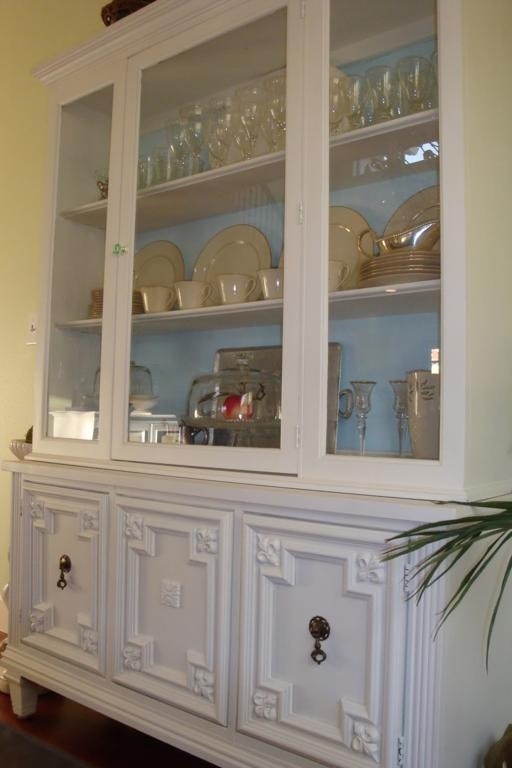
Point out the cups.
[135,258,354,315]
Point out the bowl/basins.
[129,393,158,410]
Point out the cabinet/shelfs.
[0,1,512,762]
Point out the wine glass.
[128,51,431,190]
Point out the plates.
[83,283,142,323]
[119,235,186,318]
[192,221,274,308]
[279,202,376,292]
[357,179,442,291]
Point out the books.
[51,408,181,445]
